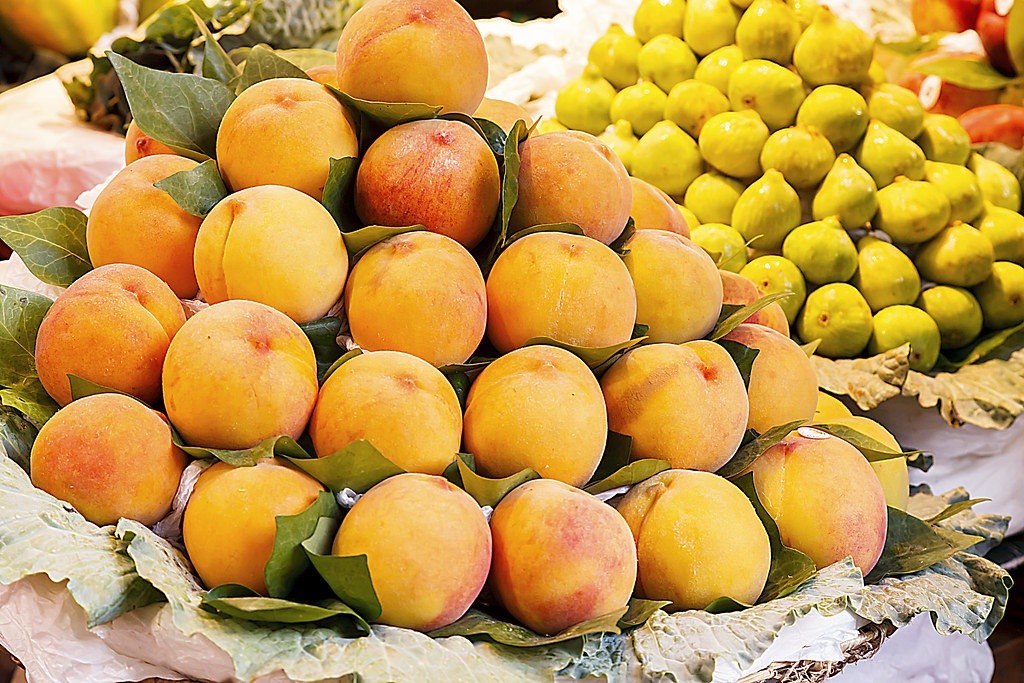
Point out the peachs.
[26,0,908,632]
[894,0,1024,157]
